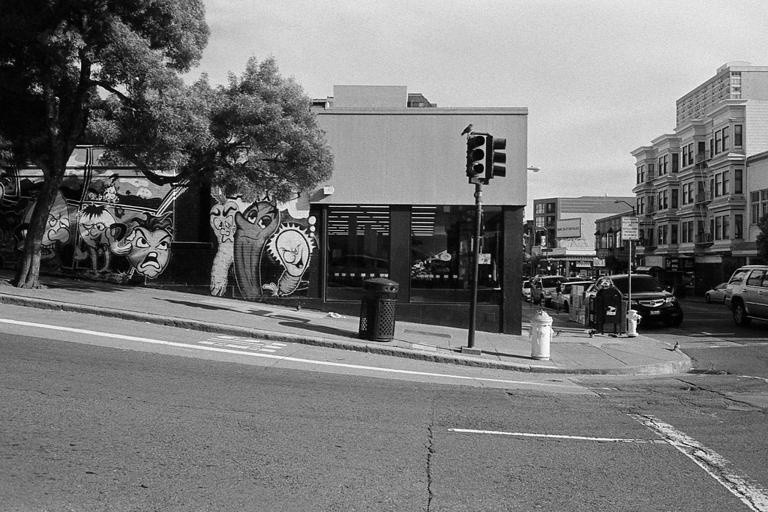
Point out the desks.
[613,199,635,216]
[527,163,541,174]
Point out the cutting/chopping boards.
[528,309,554,360]
[625,310,641,336]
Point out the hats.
[705,282,728,305]
[521,272,683,331]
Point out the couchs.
[466,136,484,179]
[486,133,507,183]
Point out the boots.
[724,265,768,330]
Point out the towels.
[593,284,623,336]
[359,278,400,342]
